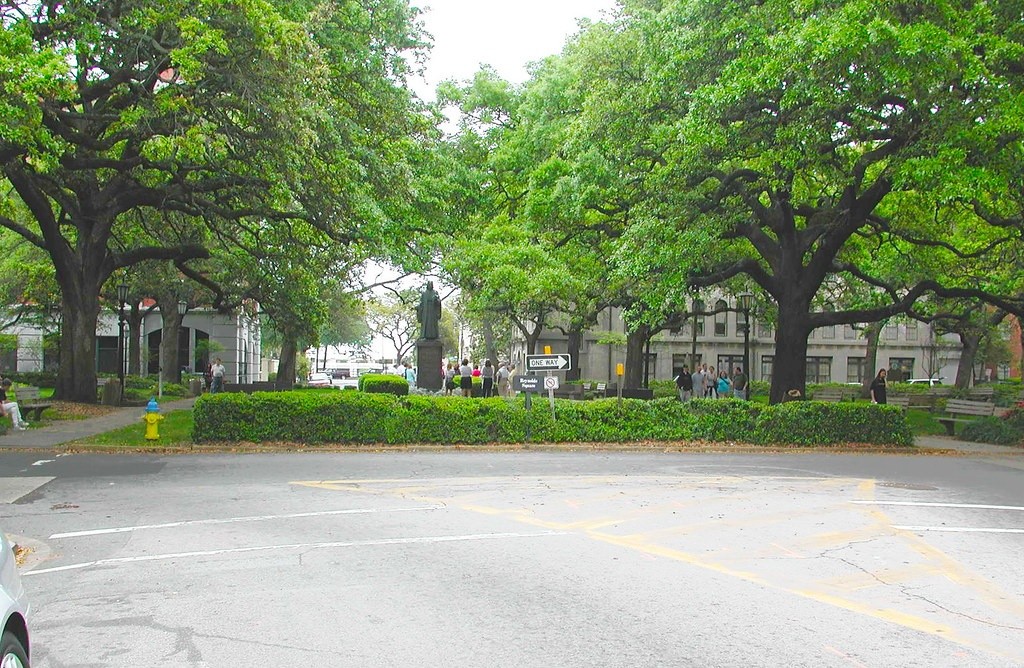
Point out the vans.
[321,368,350,380]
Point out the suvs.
[906,378,942,387]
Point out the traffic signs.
[525,353,572,372]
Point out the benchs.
[582,382,606,398]
[811,388,843,403]
[901,384,993,412]
[935,398,996,436]
[13,386,51,421]
[96,377,107,392]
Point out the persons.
[210,358,226,394]
[871,369,887,404]
[203,363,213,392]
[392,361,416,386]
[733,367,748,401]
[415,281,441,342]
[445,359,518,397]
[0,379,30,431]
[677,363,733,403]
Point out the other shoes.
[14,428,25,431]
[18,423,29,426]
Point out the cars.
[360,369,383,376]
[308,373,330,386]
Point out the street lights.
[117,278,131,393]
[644,320,652,389]
[176,299,188,348]
[740,285,754,401]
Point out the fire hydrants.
[141,396,164,441]
